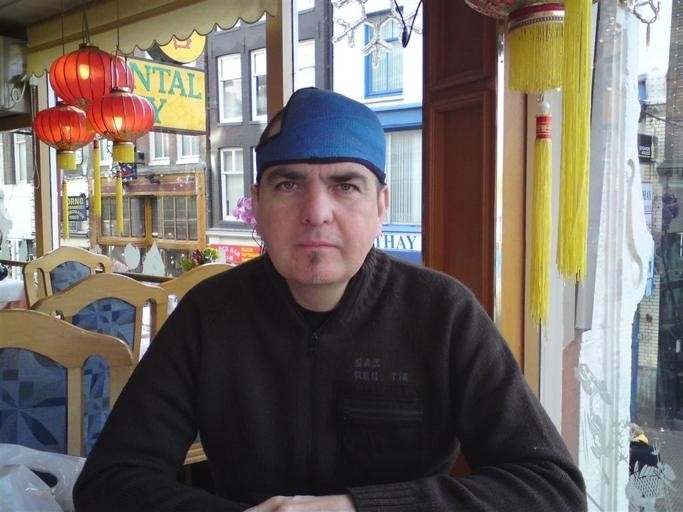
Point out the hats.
[255,87,387,187]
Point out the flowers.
[230,197,265,255]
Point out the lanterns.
[48,42,134,216]
[34,102,93,239]
[87,82,156,234]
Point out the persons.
[628,422,662,512]
[72,86,587,511]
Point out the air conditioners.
[0,36,30,119]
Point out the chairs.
[20,245,111,310]
[156,262,236,306]
[0,308,135,458]
[32,272,169,365]
